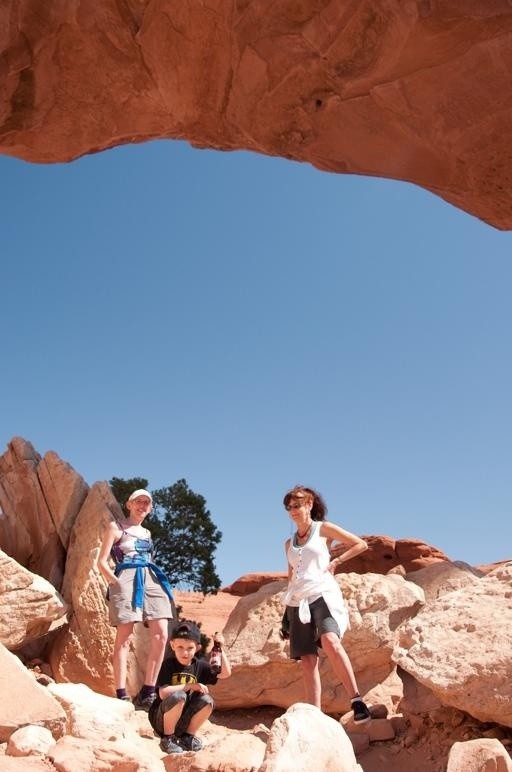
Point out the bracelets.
[330,561,337,568]
[334,557,343,565]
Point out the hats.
[171,622,201,643]
[128,489,153,503]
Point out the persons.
[281,486,371,725]
[149,623,231,754]
[97,488,173,711]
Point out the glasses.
[285,502,307,511]
[132,500,151,505]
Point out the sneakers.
[120,693,156,712]
[159,734,202,755]
[351,696,371,725]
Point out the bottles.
[211,633,222,675]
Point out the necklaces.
[296,519,314,538]
